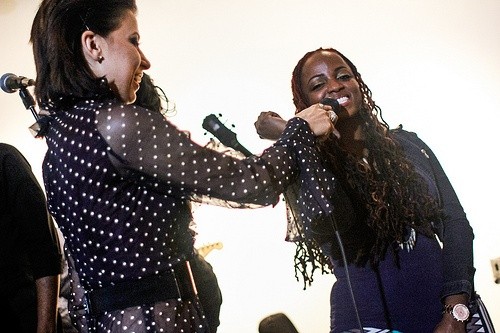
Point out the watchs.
[442,302,472,324]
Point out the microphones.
[0,73,33,93]
[321,98,340,114]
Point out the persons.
[29,1,343,333]
[254,47,494,333]
[0,142,65,333]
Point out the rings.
[319,102,324,109]
[328,109,338,122]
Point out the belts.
[86,258,208,316]
